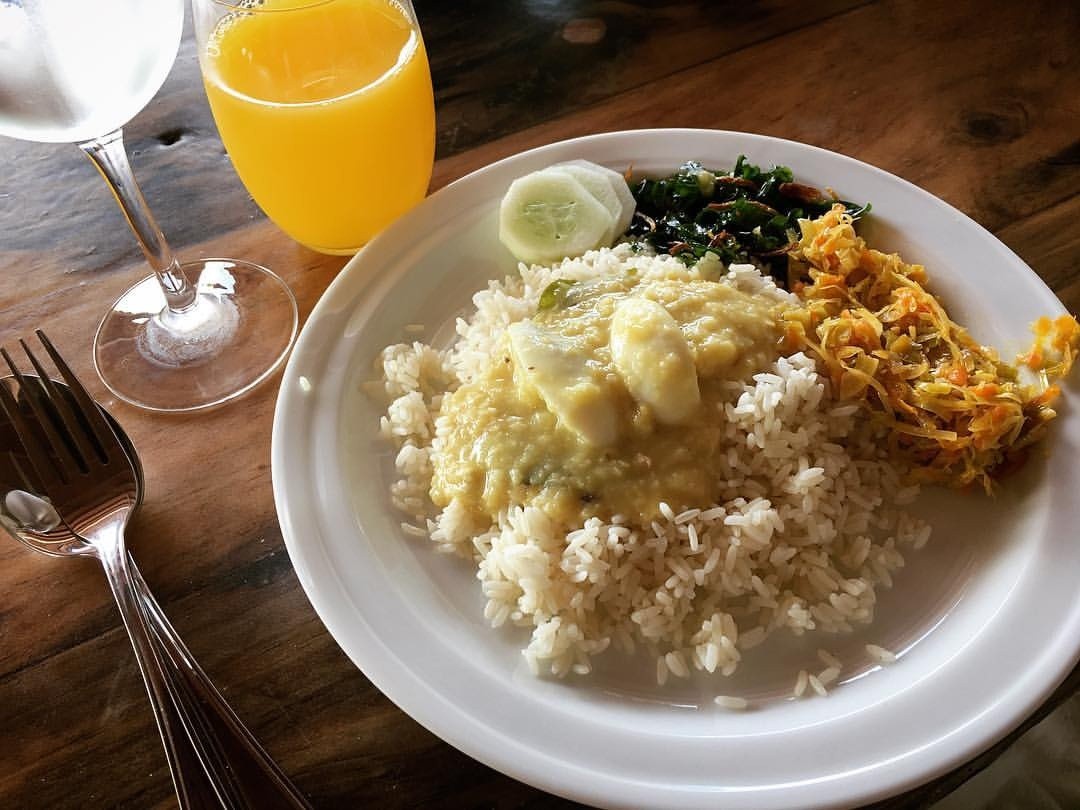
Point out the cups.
[190,0,440,262]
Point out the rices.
[349,223,948,722]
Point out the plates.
[273,124,1080,806]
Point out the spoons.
[0,372,314,810]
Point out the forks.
[0,321,239,808]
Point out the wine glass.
[0,0,299,412]
